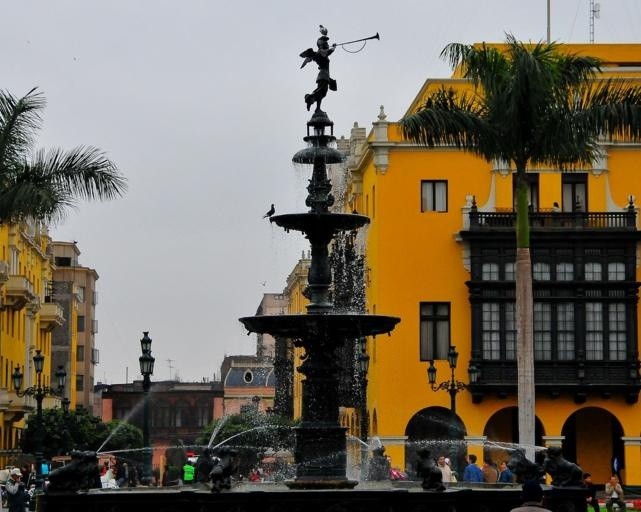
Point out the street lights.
[11,347,67,494]
[138,331,157,482]
[426,344,482,417]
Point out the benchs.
[586,502,634,512]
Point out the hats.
[11,468,23,476]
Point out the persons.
[298,36,337,111]
[369,444,553,489]
[604,474,626,512]
[5,466,30,512]
[583,473,600,512]
[19,464,30,485]
[509,482,554,512]
[98,449,294,489]
